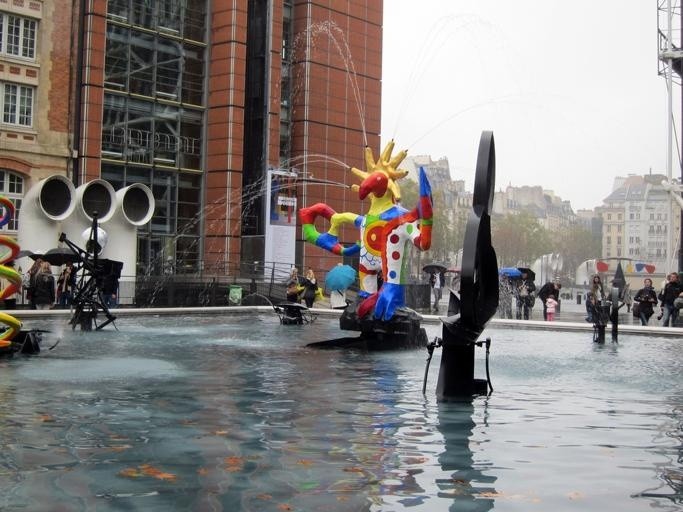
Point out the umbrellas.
[27,254,62,266]
[445,266,461,273]
[43,248,83,263]
[14,250,33,258]
[422,263,447,274]
[324,264,357,290]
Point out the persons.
[278,266,298,289]
[32,261,57,309]
[57,261,78,307]
[329,290,349,310]
[26,258,44,309]
[5,261,24,303]
[498,259,682,328]
[451,273,460,294]
[284,279,304,325]
[303,268,318,309]
[429,267,444,313]
[99,278,119,309]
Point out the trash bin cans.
[228,285,242,306]
